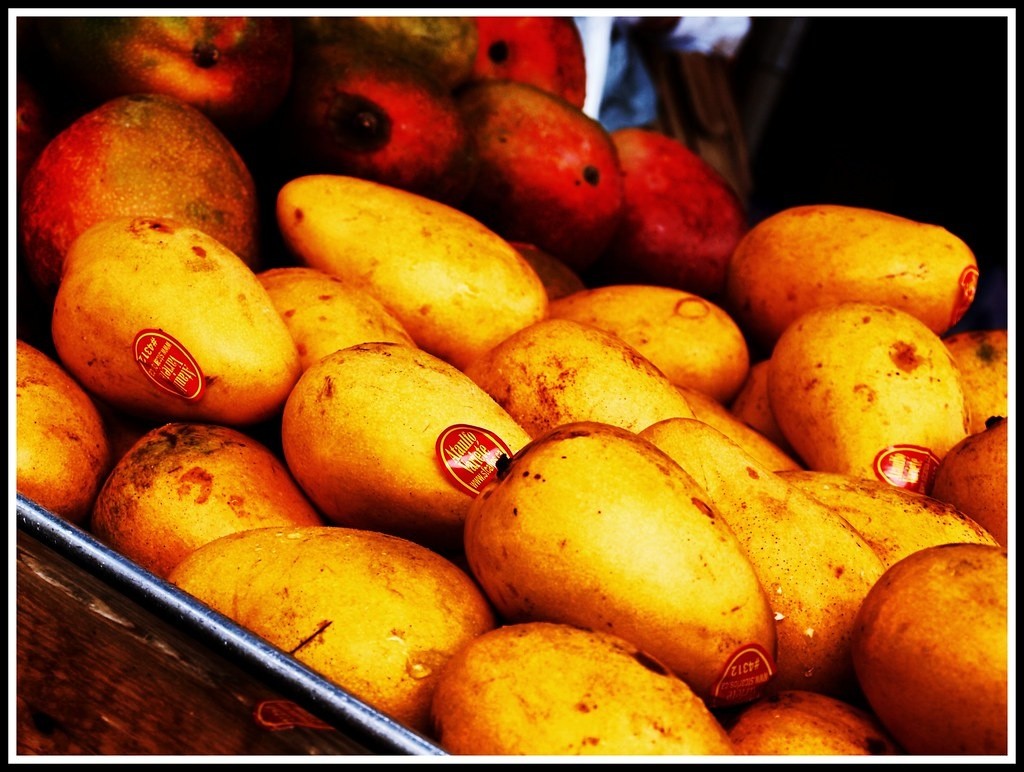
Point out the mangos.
[17,16,1008,755]
